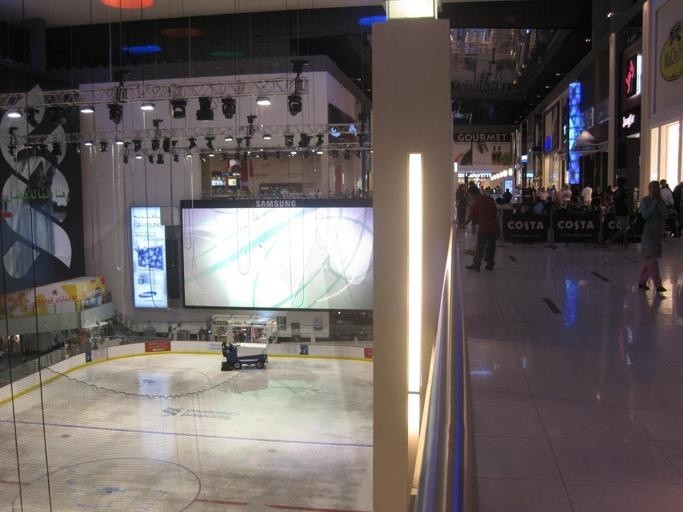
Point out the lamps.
[281,133,325,149]
[130,136,215,164]
[107,94,302,125]
[24,105,40,127]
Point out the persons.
[455,185,499,271]
[456,178,682,248]
[638,180,668,292]
[232,330,250,343]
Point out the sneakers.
[638,284,667,293]
[463,262,494,272]
